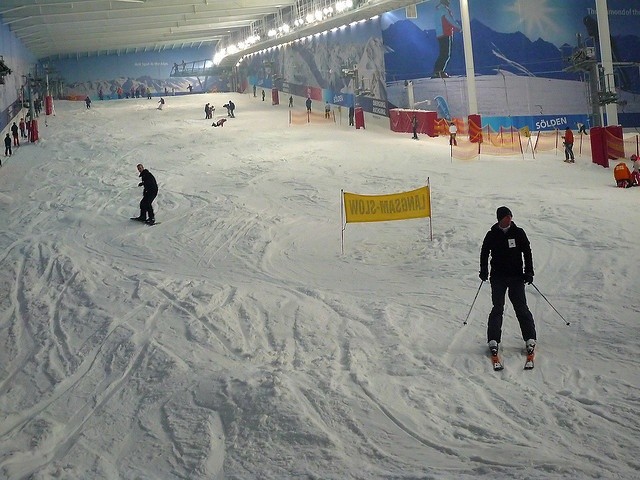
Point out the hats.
[497,206,513,222]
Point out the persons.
[33,97,41,118]
[253,85,256,97]
[306,97,312,113]
[172,63,179,76]
[630,154,639,186]
[211,119,227,128]
[4,133,12,157]
[146,88,151,99]
[324,100,331,119]
[136,88,139,98]
[137,164,158,224]
[164,88,169,96]
[449,122,458,146]
[561,126,575,163]
[209,106,215,119]
[205,103,210,119]
[262,90,265,102]
[614,162,632,187]
[574,121,588,135]
[19,118,27,139]
[85,97,91,109]
[479,206,537,371]
[11,121,20,147]
[229,100,235,118]
[180,60,186,75]
[289,95,294,107]
[223,104,231,116]
[98,89,103,100]
[157,98,164,110]
[25,113,31,130]
[410,114,418,140]
[117,87,123,99]
[140,85,146,98]
[187,84,193,94]
[430,0,463,78]
[131,87,135,99]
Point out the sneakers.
[526,339,536,354]
[489,340,498,356]
[145,218,155,224]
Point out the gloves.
[479,271,489,281]
[522,271,534,285]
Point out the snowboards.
[130,215,160,225]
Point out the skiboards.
[489,340,536,373]
